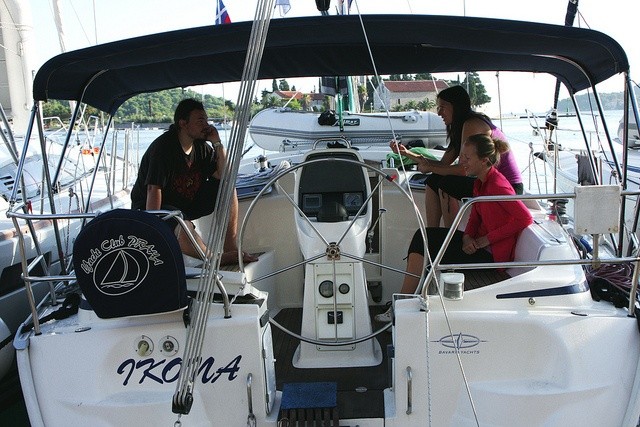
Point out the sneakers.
[374,300,393,322]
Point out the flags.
[215,0,232,24]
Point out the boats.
[534,109,639,266]
[247,0,476,152]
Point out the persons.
[131,99,259,266]
[374,133,533,331]
[389,85,523,230]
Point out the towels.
[386,146,436,170]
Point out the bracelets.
[212,140,221,147]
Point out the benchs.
[419,199,543,294]
[167,207,276,322]
